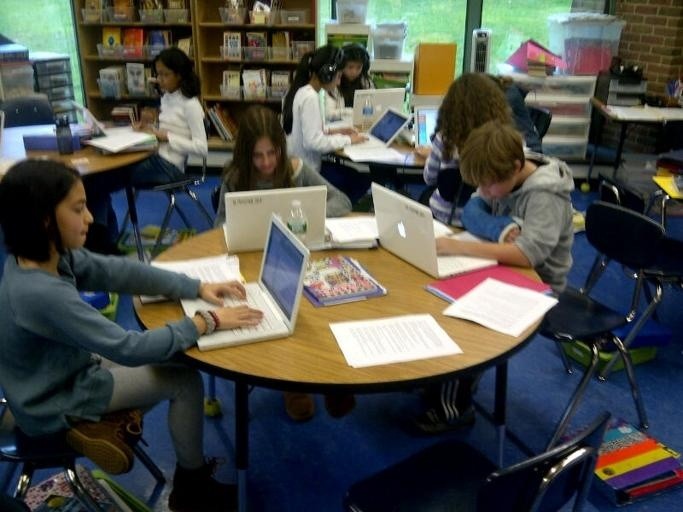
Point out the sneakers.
[413,407,475,435]
[168,457,238,512]
[325,396,355,419]
[283,393,315,421]
[67,409,143,474]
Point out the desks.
[334,141,426,193]
[588,97,683,180]
[133,211,546,512]
[0,122,158,262]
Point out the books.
[140,253,246,303]
[207,104,237,140]
[78,127,159,155]
[271,70,290,98]
[139,0,164,23]
[424,265,555,304]
[301,255,386,309]
[167,0,187,23]
[112,0,134,20]
[324,214,380,251]
[246,32,267,61]
[98,64,125,97]
[126,63,154,95]
[223,31,243,61]
[84,0,112,19]
[240,68,270,100]
[111,103,138,126]
[222,65,243,100]
[124,28,145,56]
[149,29,173,55]
[101,27,122,50]
[272,30,290,61]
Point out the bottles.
[55,127,73,155]
[286,200,307,242]
[362,96,374,130]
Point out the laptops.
[222,185,327,254]
[343,106,411,151]
[180,211,310,352]
[341,87,406,127]
[371,182,498,279]
[414,104,441,158]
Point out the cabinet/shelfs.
[513,73,594,163]
[29,50,75,124]
[70,0,318,152]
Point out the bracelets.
[194,309,219,335]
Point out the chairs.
[114,119,212,257]
[435,166,474,224]
[0,93,57,128]
[341,410,612,512]
[0,398,163,511]
[528,105,552,141]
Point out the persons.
[278,45,369,207]
[416,121,575,437]
[337,45,375,107]
[84,48,209,258]
[0,156,263,512]
[417,72,513,230]
[488,74,543,153]
[215,105,353,226]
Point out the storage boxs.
[547,12,626,76]
[325,0,407,59]
